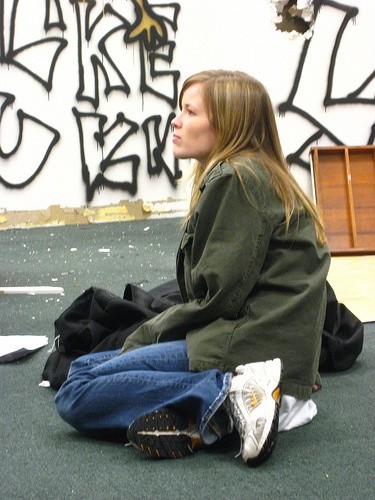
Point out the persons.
[54,70,333,468]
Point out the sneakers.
[127,409,202,457]
[226,358,281,466]
